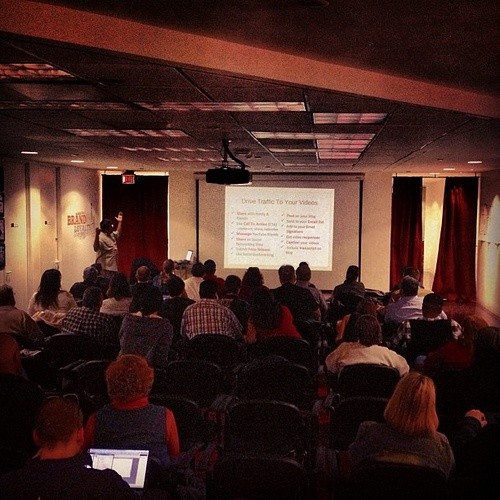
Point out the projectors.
[206,168,252,185]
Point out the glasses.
[43,394,80,426]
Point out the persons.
[0,259,500,378]
[0,400,141,500]
[452,407,500,480]
[347,373,455,480]
[94,211,124,282]
[83,354,179,483]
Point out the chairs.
[0,290,500,500]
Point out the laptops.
[186,250,193,262]
[85,446,151,498]
